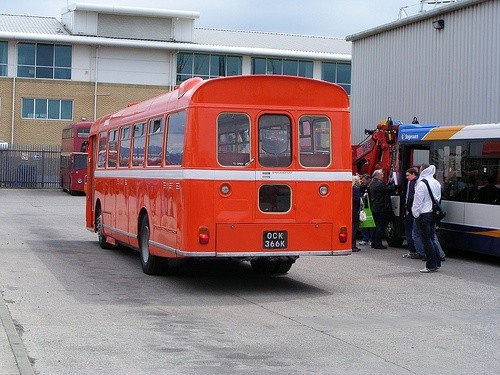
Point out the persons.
[411,162,443,273]
[399,166,446,262]
[351,169,398,251]
[431,171,500,206]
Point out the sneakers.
[419,266,440,272]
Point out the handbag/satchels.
[359,194,377,228]
[433,200,446,221]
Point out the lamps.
[81,117,87,120]
[432,20,444,30]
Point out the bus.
[82,72,358,276]
[57,121,97,196]
[392,121,500,261]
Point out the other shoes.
[404,252,445,261]
[353,240,387,251]
